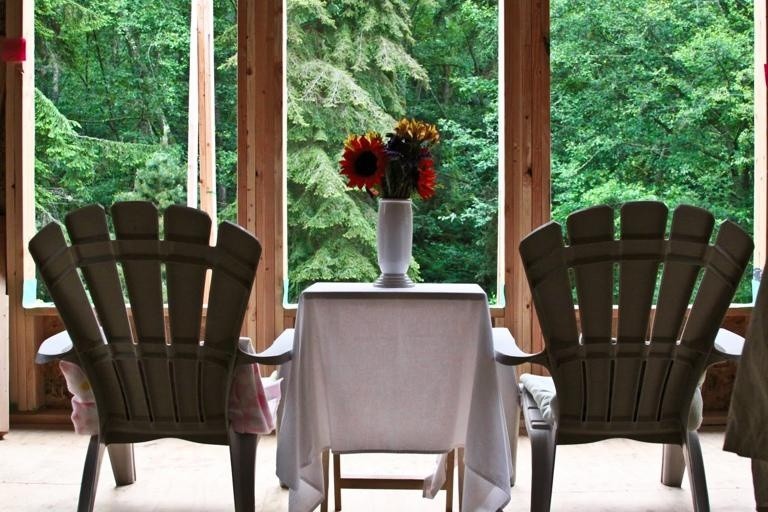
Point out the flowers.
[338,118,441,199]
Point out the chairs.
[26,201,295,512]
[491,200,749,512]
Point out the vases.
[373,197,414,287]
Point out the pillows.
[58,338,273,435]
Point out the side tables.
[276,282,519,512]
